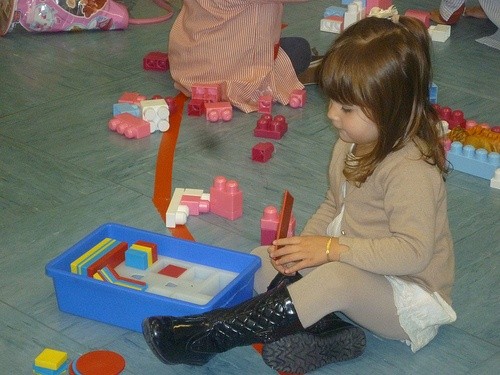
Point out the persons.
[142,4,457,374]
[427,0,500,51]
[167,0,327,113]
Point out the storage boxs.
[46,223,262,335]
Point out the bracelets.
[326,236,334,262]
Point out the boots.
[262,270,366,374]
[141,279,305,366]
